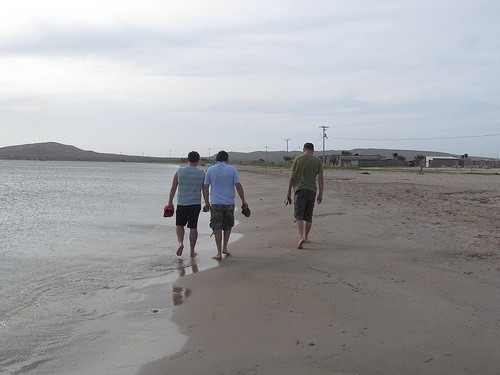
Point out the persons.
[287,142,323,248]
[168,150,211,256]
[203,150,248,258]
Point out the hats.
[163,205,174,217]
